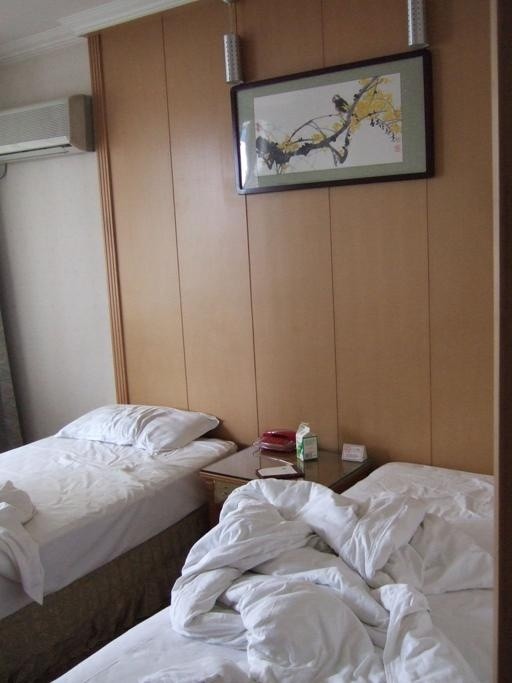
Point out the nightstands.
[197,447,376,529]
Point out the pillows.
[52,404,220,458]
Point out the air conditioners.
[0,95,96,164]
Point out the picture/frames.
[229,49,434,196]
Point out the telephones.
[259,429,296,450]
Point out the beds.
[0,433,239,683]
[40,456,41,457]
[51,459,498,683]
